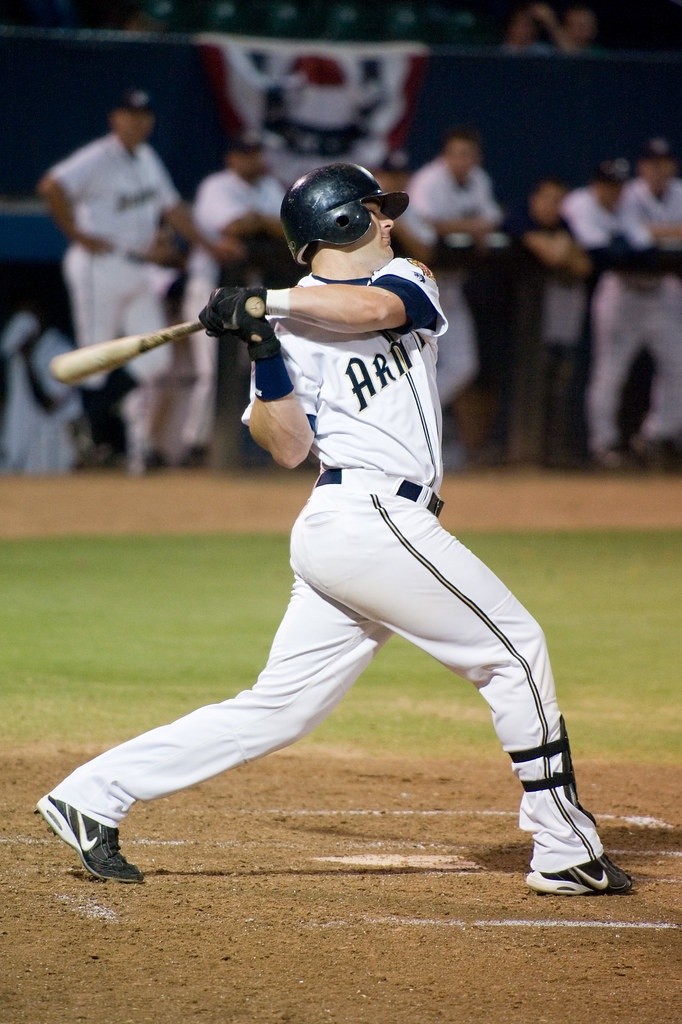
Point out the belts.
[316,468,445,518]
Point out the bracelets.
[264,288,291,318]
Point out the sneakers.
[37,794,144,884]
[525,853,633,895]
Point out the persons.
[33,162,637,898]
[37,87,681,480]
[508,0,596,52]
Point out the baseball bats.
[50,296,264,384]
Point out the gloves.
[198,286,248,338]
[218,291,281,360]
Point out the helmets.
[279,161,409,266]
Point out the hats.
[598,156,630,181]
[637,136,677,161]
[227,134,263,154]
[109,85,160,114]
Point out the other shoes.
[595,441,631,468]
[144,453,166,472]
[636,434,680,472]
[182,444,209,468]
